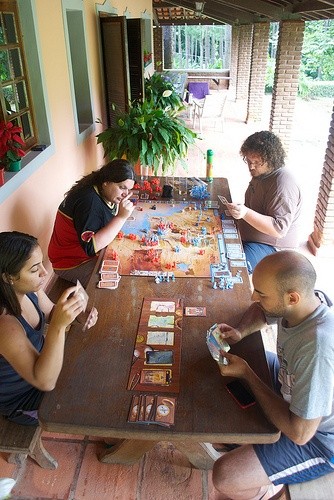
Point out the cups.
[140,165,149,182]
[166,175,175,192]
[178,176,188,195]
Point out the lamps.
[194,0,207,17]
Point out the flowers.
[0,118,27,168]
[144,50,153,68]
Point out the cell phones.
[224,380,256,408]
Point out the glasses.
[242,156,265,167]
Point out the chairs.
[183,89,230,134]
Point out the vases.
[8,160,22,171]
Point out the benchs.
[261,321,334,500]
[0,413,59,470]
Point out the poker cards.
[221,219,248,268]
[96,260,121,291]
[206,323,231,366]
[140,369,172,386]
[148,300,176,312]
[147,315,175,329]
[218,195,229,205]
[72,278,95,332]
[145,331,175,346]
[145,350,174,365]
[184,306,207,317]
[224,210,231,216]
[128,394,177,426]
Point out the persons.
[48,158,137,288]
[0,231,98,425]
[226,131,302,275]
[211,250,334,500]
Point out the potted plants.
[92,61,207,179]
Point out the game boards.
[99,200,232,279]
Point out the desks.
[36,177,282,469]
